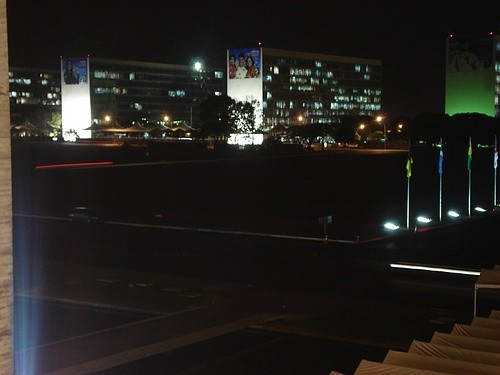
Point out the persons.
[246,57,258,78]
[64,61,79,85]
[230,55,238,79]
[237,54,249,79]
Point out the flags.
[437,143,444,175]
[405,160,412,179]
[493,148,499,169]
[466,143,474,172]
